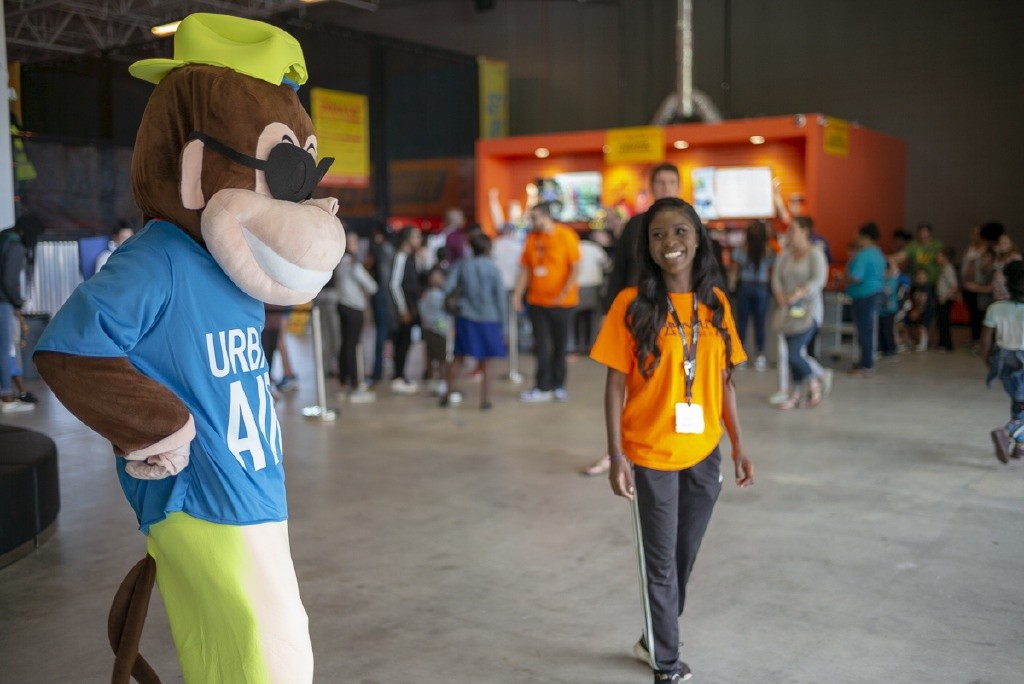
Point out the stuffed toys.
[29,12,347,684]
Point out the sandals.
[584,455,611,476]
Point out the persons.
[94,220,133,278]
[977,258,1023,462]
[7,310,39,405]
[568,214,1023,409]
[511,203,579,403]
[589,196,756,684]
[250,219,528,413]
[581,161,732,488]
[0,203,26,398]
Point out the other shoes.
[783,392,801,410]
[821,367,835,400]
[338,371,493,411]
[805,382,821,408]
[767,389,790,402]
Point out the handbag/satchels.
[774,303,813,335]
[443,284,467,315]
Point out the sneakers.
[993,430,1011,463]
[20,392,37,403]
[278,373,300,391]
[0,398,35,413]
[557,388,566,400]
[633,635,691,680]
[520,385,551,402]
[1012,445,1024,460]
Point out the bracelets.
[610,454,626,462]
